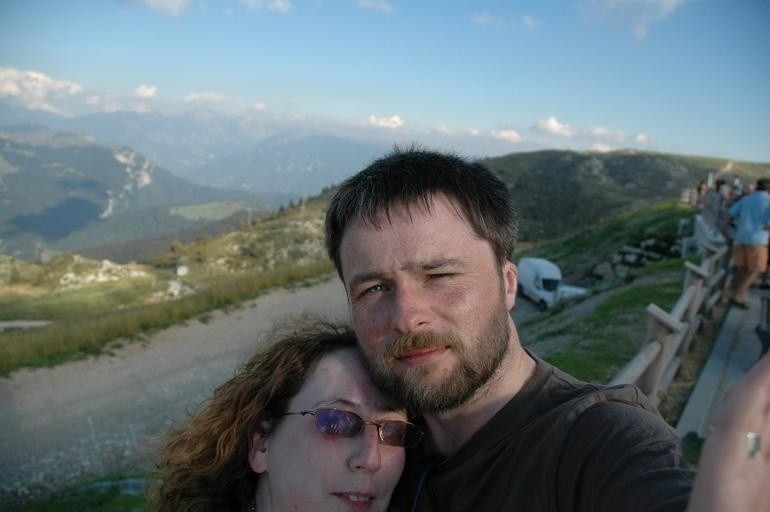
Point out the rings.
[704,424,762,459]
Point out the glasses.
[281,407,424,446]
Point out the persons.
[698,179,769,311]
[143,314,427,512]
[321,142,770,512]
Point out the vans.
[516,255,563,311]
